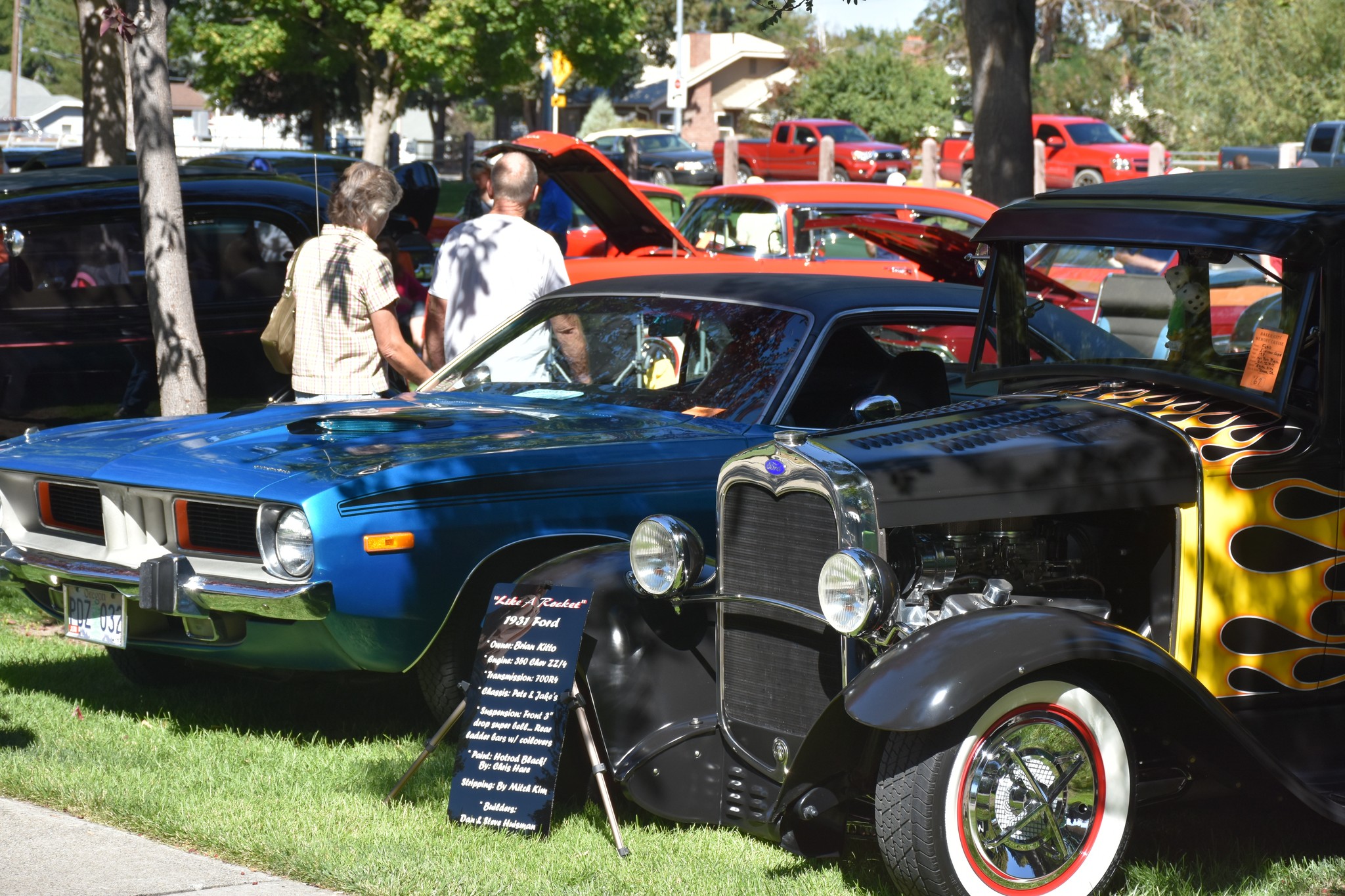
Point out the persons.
[864,172,922,260]
[457,160,493,219]
[1232,154,1249,172]
[1116,166,1195,279]
[374,236,425,314]
[535,165,569,258]
[260,161,435,405]
[736,176,777,256]
[424,151,592,384]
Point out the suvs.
[504,169,1344,895]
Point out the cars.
[0,128,1248,740]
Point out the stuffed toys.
[1159,266,1208,362]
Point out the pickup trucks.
[712,115,1345,196]
